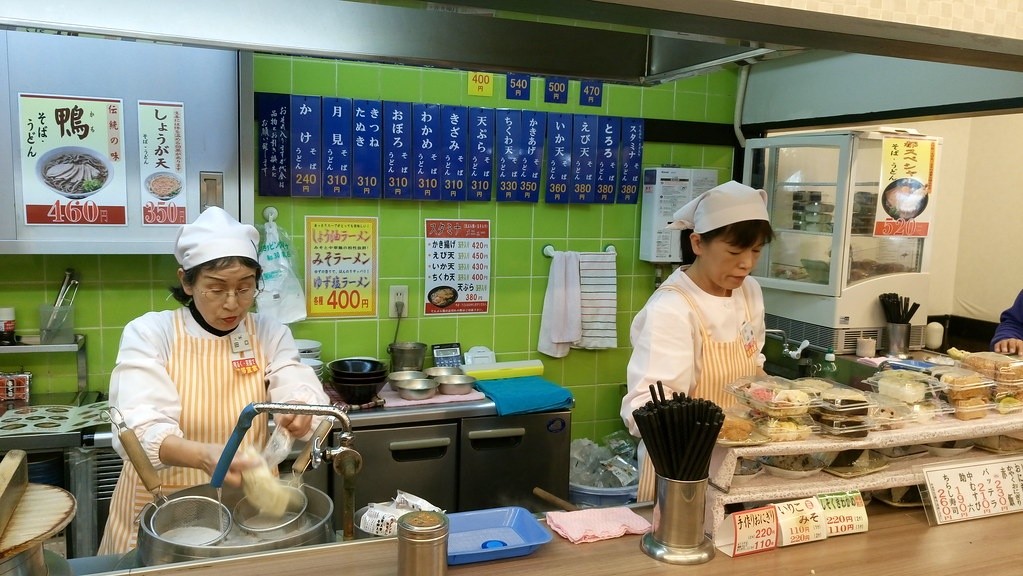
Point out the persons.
[619,181,779,505]
[96,207,331,558]
[990,289,1023,356]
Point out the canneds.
[0,308,15,344]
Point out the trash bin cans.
[568,475,641,510]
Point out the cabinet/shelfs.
[330,413,571,531]
[702,402,1022,531]
[0,22,256,255]
[743,132,941,354]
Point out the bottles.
[396,510,449,576]
[0,307,16,331]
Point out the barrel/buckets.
[387,341,428,372]
[568,481,639,509]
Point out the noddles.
[42,154,108,194]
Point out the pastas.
[147,174,181,196]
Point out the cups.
[39,305,75,345]
[855,338,877,358]
[640,472,716,565]
[885,323,912,355]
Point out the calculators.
[432,343,466,367]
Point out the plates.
[0,403,110,430]
[759,459,825,479]
[974,435,1023,455]
[872,493,931,508]
[821,463,891,479]
[716,432,772,447]
[732,457,765,483]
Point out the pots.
[135,476,339,567]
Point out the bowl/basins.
[293,339,390,404]
[882,178,929,222]
[423,365,463,377]
[35,145,115,200]
[921,444,976,457]
[353,501,395,539]
[869,448,929,461]
[394,377,440,400]
[444,505,555,565]
[144,172,185,201]
[388,369,428,391]
[728,351,1023,439]
[435,374,476,395]
[427,286,458,308]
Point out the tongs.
[45,280,80,344]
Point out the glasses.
[190,280,260,300]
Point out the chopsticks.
[632,381,726,481]
[879,293,921,324]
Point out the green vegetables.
[82,179,102,192]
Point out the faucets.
[765,329,790,347]
